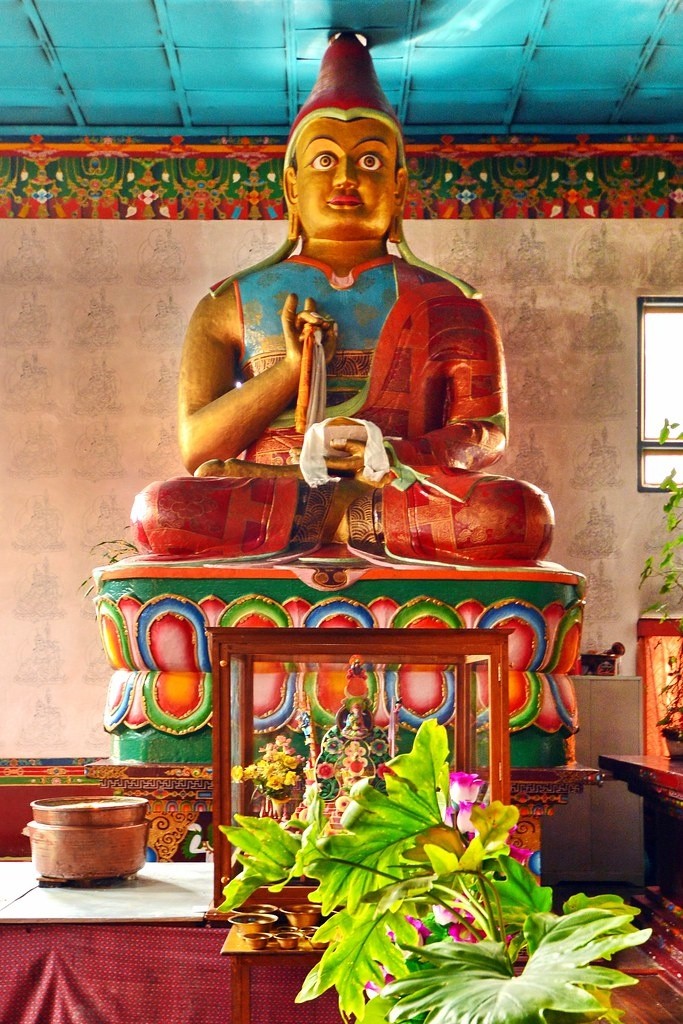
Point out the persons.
[131,32,558,569]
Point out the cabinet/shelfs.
[541,676,645,886]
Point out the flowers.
[216,717,653,1024]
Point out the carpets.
[607,931,667,975]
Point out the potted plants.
[637,418,683,758]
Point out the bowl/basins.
[226,903,335,951]
[27,797,150,877]
[581,654,620,675]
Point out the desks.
[598,755,683,991]
[84,761,612,886]
[0,861,345,1024]
[221,925,330,1024]
